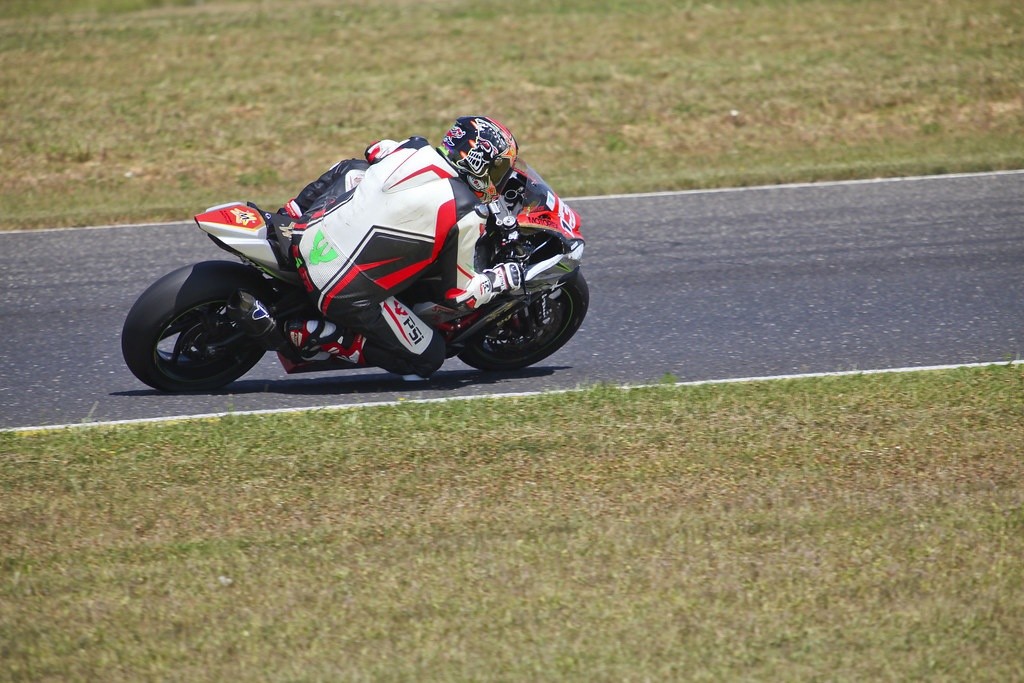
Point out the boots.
[283,318,368,366]
[276,199,302,219]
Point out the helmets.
[436,115,520,205]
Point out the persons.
[277,116,525,377]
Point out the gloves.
[455,262,522,308]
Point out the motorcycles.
[113,137,590,396]
